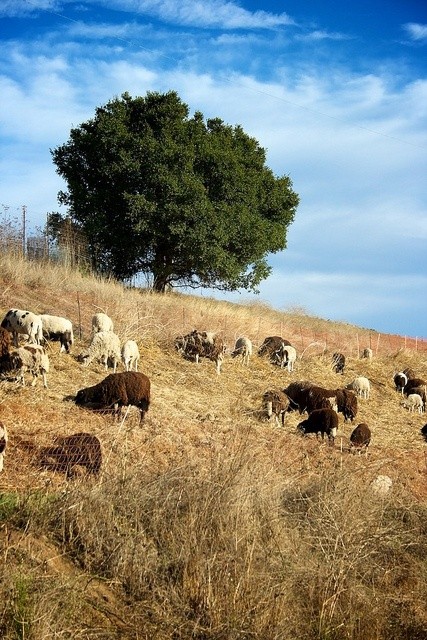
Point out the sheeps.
[174,330,226,375]
[121,340,139,373]
[272,344,296,372]
[363,349,372,359]
[393,372,407,397]
[348,423,372,457]
[347,377,370,398]
[73,370,150,429]
[37,315,73,354]
[0,308,42,347]
[91,313,113,333]
[403,378,426,394]
[296,408,338,446]
[406,387,425,404]
[327,389,357,422]
[230,336,253,366]
[283,381,337,421]
[0,343,50,389]
[258,336,291,359]
[401,394,423,414]
[75,332,121,374]
[403,368,415,380]
[41,432,101,478]
[262,390,290,427]
[331,353,345,375]
